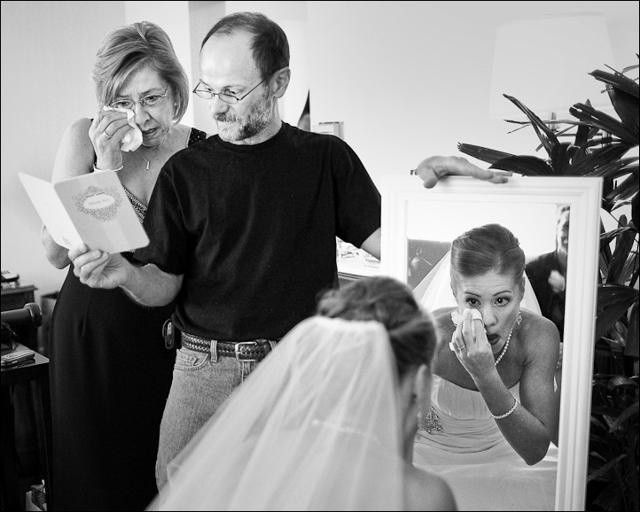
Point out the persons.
[144,275,460,512]
[525,205,570,387]
[41,19,211,512]
[409,222,564,512]
[68,10,515,494]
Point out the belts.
[179,334,277,360]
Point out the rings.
[104,130,112,138]
[458,345,465,351]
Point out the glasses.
[109,85,175,110]
[190,80,266,104]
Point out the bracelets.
[92,161,124,173]
[490,396,518,420]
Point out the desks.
[0,342,49,479]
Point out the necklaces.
[138,145,161,170]
[494,321,514,366]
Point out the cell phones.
[1,349,35,368]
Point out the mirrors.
[381,176,604,511]
